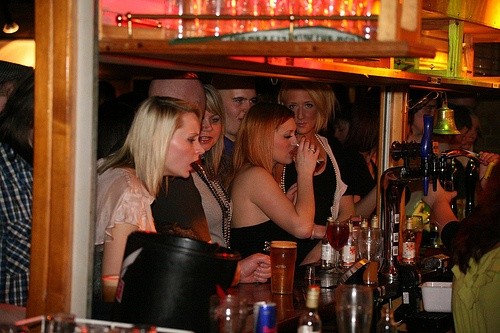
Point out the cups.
[163,0,379,41]
[357,228,382,268]
[401,214,422,265]
[362,259,380,285]
[269,241,297,294]
[334,284,373,333]
[209,294,249,333]
[288,134,326,172]
[305,266,314,280]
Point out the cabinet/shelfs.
[97,0,500,89]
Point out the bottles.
[320,215,382,273]
[402,217,415,264]
[297,286,322,333]
[255,303,278,333]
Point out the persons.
[0,69,500,333]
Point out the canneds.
[253,301,277,333]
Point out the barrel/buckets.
[107,230,242,333]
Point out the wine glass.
[325,217,350,275]
